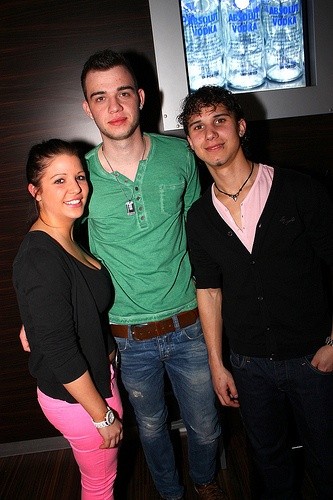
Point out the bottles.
[226,0,266,89]
[263,0,304,82]
[181,0,226,90]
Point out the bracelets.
[326,336,333,349]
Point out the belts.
[108,307,199,341]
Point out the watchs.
[92,406,116,429]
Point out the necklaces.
[103,140,147,215]
[215,161,255,199]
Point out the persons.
[178,84,333,500]
[19,53,222,500]
[13,139,125,499]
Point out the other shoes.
[195,481,227,500]
